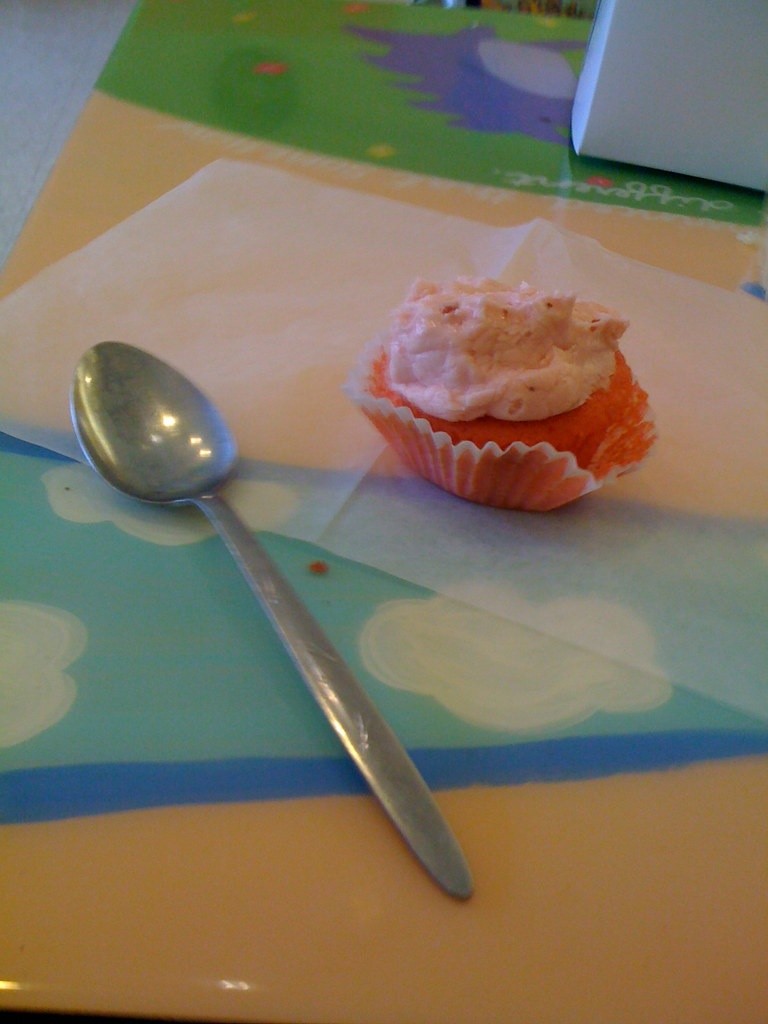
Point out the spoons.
[71,340,477,903]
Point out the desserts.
[344,273,660,512]
